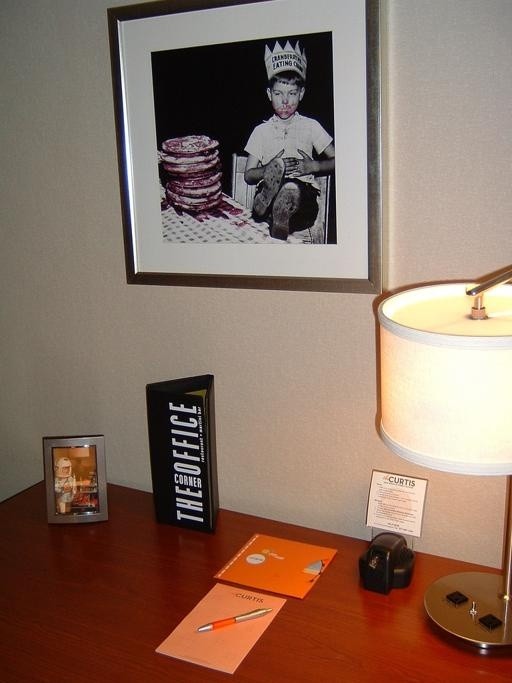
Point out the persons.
[244,69,335,241]
[53,456,77,514]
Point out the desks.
[0,479,512,682]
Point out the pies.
[162,135,222,212]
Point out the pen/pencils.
[197,608,273,633]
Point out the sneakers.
[270,182,301,240]
[254,158,285,218]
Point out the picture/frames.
[42,436,109,525]
[106,0,388,297]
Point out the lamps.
[375,266,512,651]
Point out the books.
[155,582,287,675]
[212,533,338,600]
